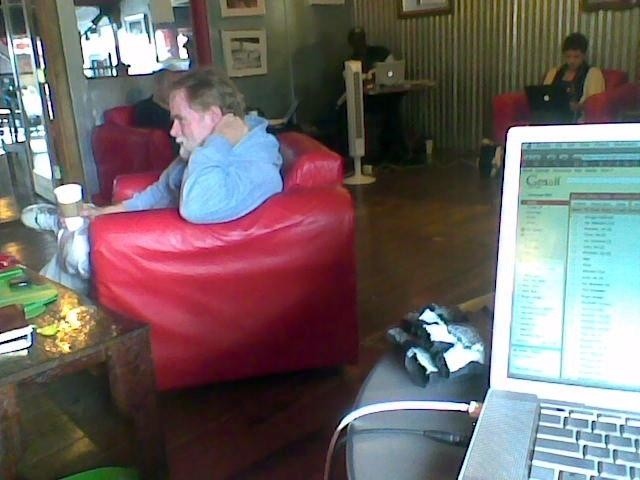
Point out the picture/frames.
[217,2,269,76]
[397,0,458,18]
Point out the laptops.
[374,60,407,88]
[524,84,574,124]
[458,123,639,479]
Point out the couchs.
[88,104,356,393]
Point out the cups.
[55,182,85,230]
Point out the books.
[0,330,35,355]
[0,304,33,345]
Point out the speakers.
[343,60,375,185]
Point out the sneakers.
[20,201,97,232]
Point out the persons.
[130,67,279,142]
[20,65,285,298]
[479,30,609,183]
[334,23,404,167]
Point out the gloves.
[383,297,489,390]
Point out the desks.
[364,80,438,167]
[345,289,495,478]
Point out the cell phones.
[9,277,30,287]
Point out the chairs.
[492,71,639,148]
